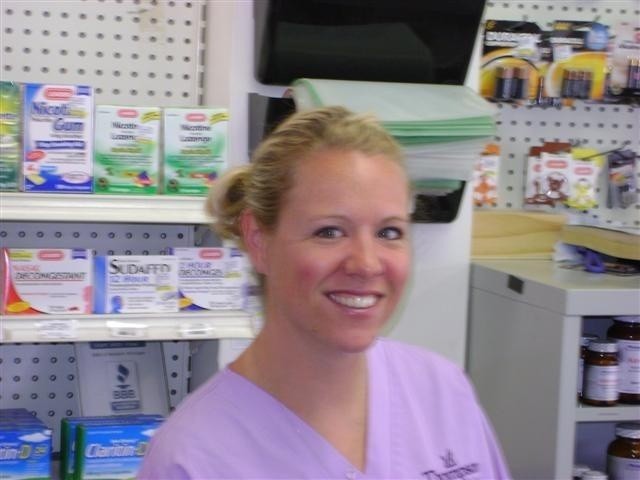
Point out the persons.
[139,108,509,479]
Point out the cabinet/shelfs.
[464,257,640,480]
[0,190,265,478]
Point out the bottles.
[576,315,640,408]
[571,421,640,479]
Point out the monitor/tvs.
[256,0,486,85]
